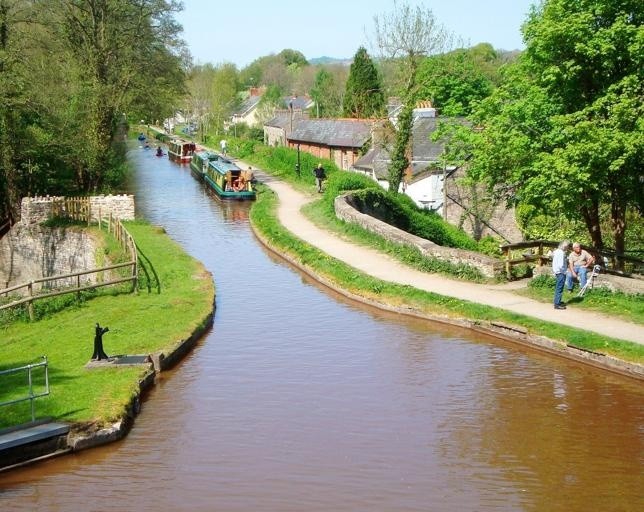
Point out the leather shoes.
[555,304,566,309]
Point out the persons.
[314,164,326,193]
[552,240,594,309]
[157,147,163,154]
[233,166,255,192]
[220,140,228,157]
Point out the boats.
[168,138,258,203]
